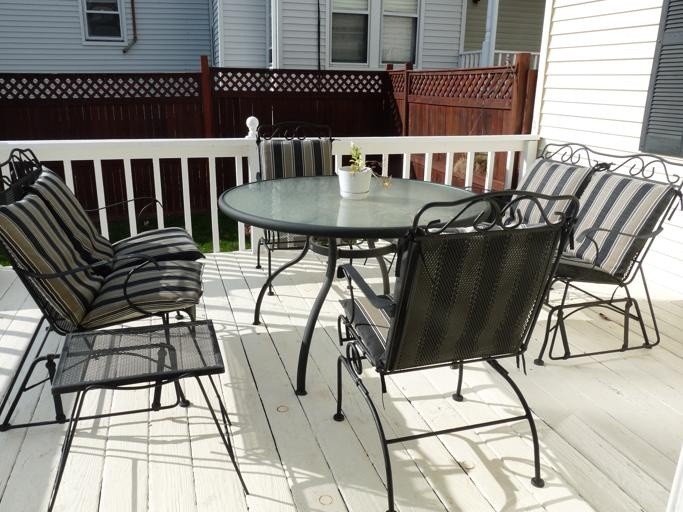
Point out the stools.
[47,319,250,512]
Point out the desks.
[218,174,492,395]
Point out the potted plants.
[338,144,394,200]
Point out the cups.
[338,166,371,199]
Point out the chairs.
[256,120,354,295]
[333,189,579,512]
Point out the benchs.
[423,143,683,366]
[0,147,206,433]
